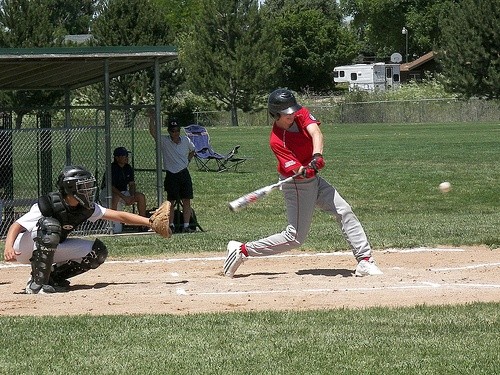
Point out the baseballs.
[439,180,451,193]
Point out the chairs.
[183,124,251,171]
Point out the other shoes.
[25,280,43,295]
[138,225,154,232]
[49,277,71,287]
[170,225,175,234]
[181,227,197,234]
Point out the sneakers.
[223,240,249,278]
[354,256,383,277]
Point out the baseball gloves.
[148,199,177,237]
[226,165,314,213]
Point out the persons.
[100,147,154,232]
[146,109,197,233]
[4,166,173,294]
[223,90,383,277]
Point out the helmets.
[267,90,302,121]
[57,165,97,193]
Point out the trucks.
[331,61,401,94]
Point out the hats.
[168,120,180,130]
[113,147,132,156]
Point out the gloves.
[298,152,325,179]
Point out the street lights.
[402,27,409,84]
